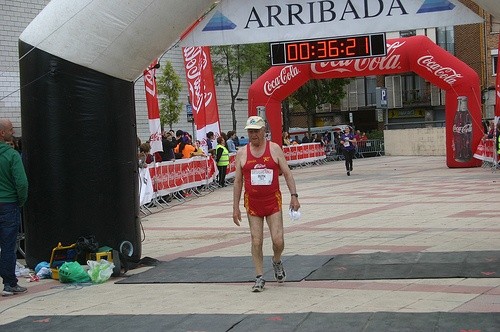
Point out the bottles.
[453,96,473,162]
[256,106,272,141]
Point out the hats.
[243,116,265,129]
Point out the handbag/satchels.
[58,261,92,283]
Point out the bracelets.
[290,193,298,197]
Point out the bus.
[289,125,353,161]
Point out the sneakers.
[1,284,28,296]
[251,276,265,292]
[271,256,286,283]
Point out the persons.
[496,117,500,151]
[206,131,215,155]
[340,126,354,176]
[136,129,207,208]
[0,118,28,296]
[215,136,229,187]
[482,120,494,139]
[233,116,300,291]
[335,130,368,160]
[222,130,244,184]
[282,130,330,170]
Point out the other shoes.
[347,172,350,176]
[350,167,353,171]
